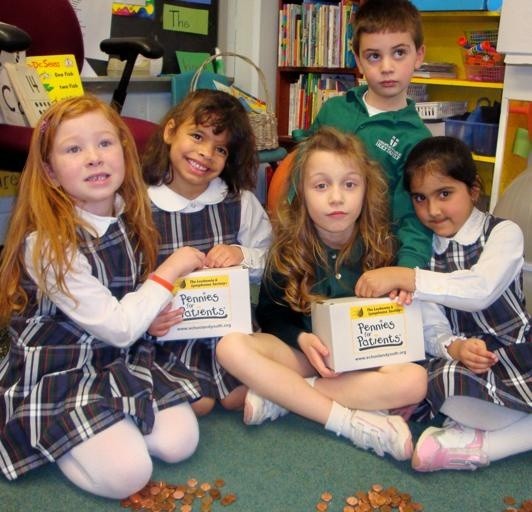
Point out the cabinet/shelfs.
[271,0,506,214]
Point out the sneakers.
[411,426,484,473]
[349,410,414,463]
[243,383,286,426]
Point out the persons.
[215,125,429,463]
[0,93,205,503]
[352,135,532,472]
[139,86,277,418]
[288,1,436,307]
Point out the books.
[276,1,468,135]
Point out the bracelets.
[148,272,173,291]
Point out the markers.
[457,36,506,82]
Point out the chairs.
[171,70,285,164]
[0,0,164,177]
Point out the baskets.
[408,84,468,121]
[191,52,280,151]
